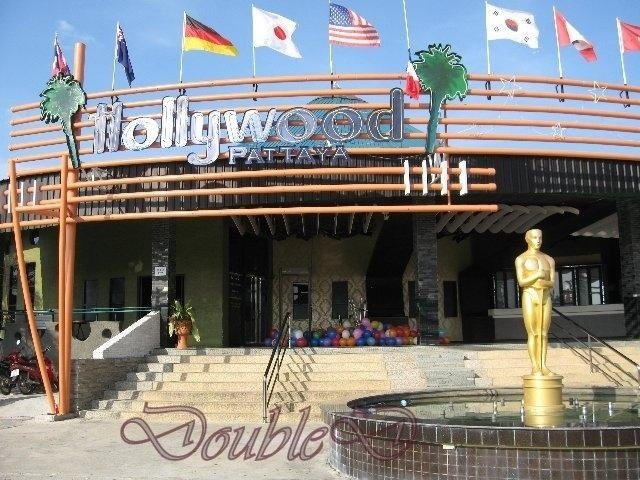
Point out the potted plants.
[167,297,201,350]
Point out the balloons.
[263,316,417,346]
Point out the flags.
[252,6,303,58]
[183,14,238,58]
[486,2,539,48]
[330,2,381,48]
[403,48,421,101]
[50,39,73,78]
[619,19,640,53]
[117,25,135,88]
[553,8,597,66]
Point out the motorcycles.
[8,345,60,396]
[0,338,29,395]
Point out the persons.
[514,227,555,377]
